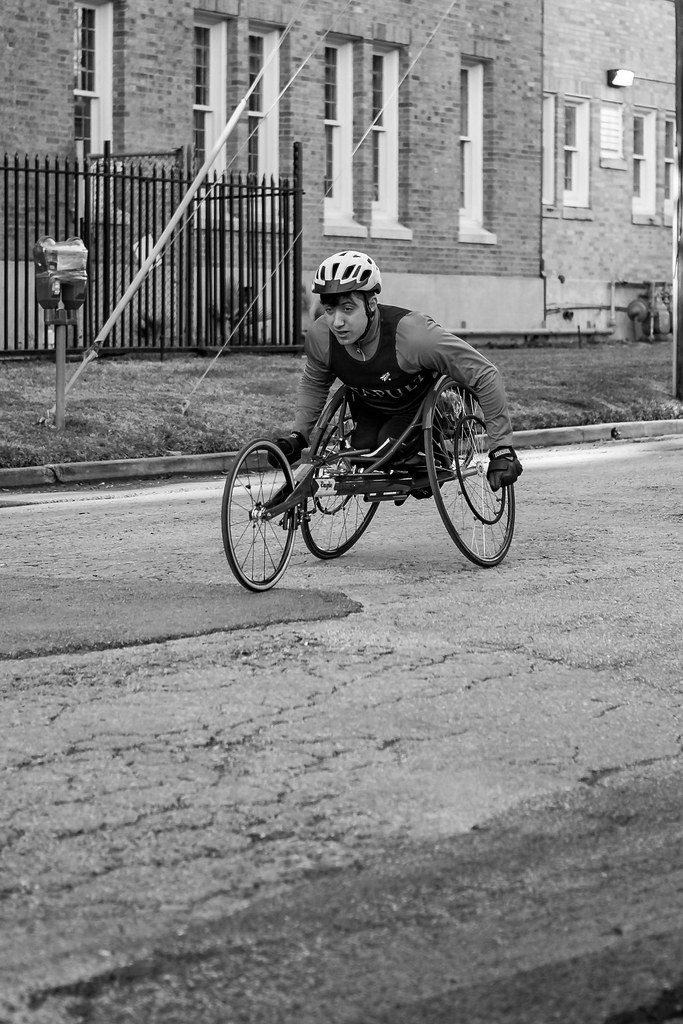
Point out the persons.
[266,251,522,491]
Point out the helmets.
[312,251,382,294]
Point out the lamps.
[608,69,636,87]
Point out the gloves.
[487,446,523,492]
[268,431,309,469]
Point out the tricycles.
[222,378,515,592]
